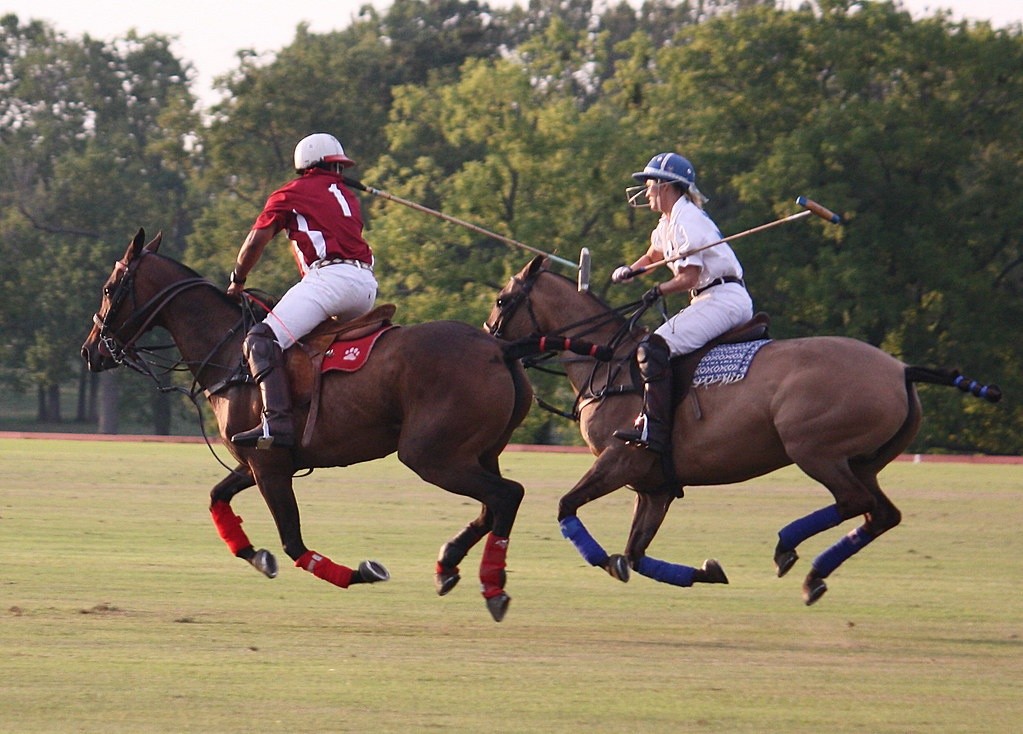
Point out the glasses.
[323,163,344,174]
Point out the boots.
[230,322,296,446]
[613,333,671,455]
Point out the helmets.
[294,133,356,169]
[631,152,696,185]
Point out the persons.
[612,152,755,456]
[227,134,379,447]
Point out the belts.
[319,258,375,272]
[688,276,743,298]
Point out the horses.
[480,245,1003,606]
[80,224,612,627]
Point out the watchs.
[230,271,246,284]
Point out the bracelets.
[625,265,633,272]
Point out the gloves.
[611,265,634,283]
[641,287,659,305]
